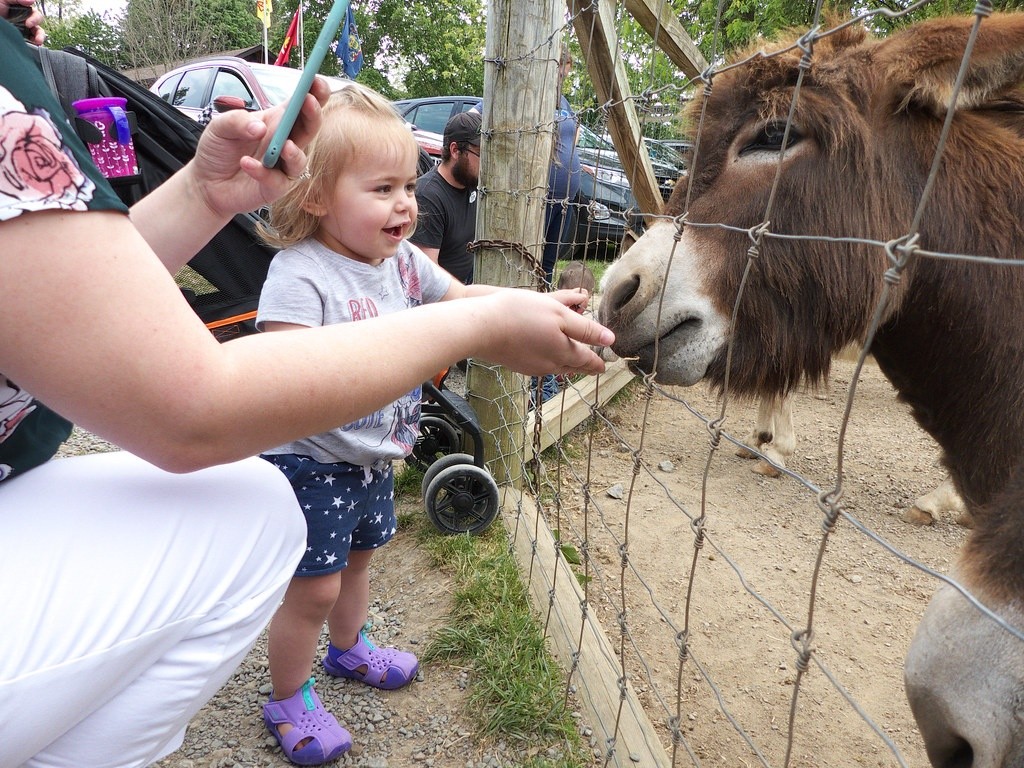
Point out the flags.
[336,4,364,80]
[256,0,273,27]
[272,7,299,67]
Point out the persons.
[413,113,480,404]
[465,47,577,387]
[0,0,617,768]
[529,261,594,414]
[251,85,591,768]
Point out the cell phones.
[262,0,349,168]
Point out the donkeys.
[593,14,1024,767]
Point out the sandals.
[321,631,418,689]
[260,678,352,766]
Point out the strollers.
[21,47,499,542]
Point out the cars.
[386,95,695,258]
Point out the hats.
[444,111,481,146]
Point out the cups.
[72,97,142,186]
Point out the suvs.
[147,55,353,128]
